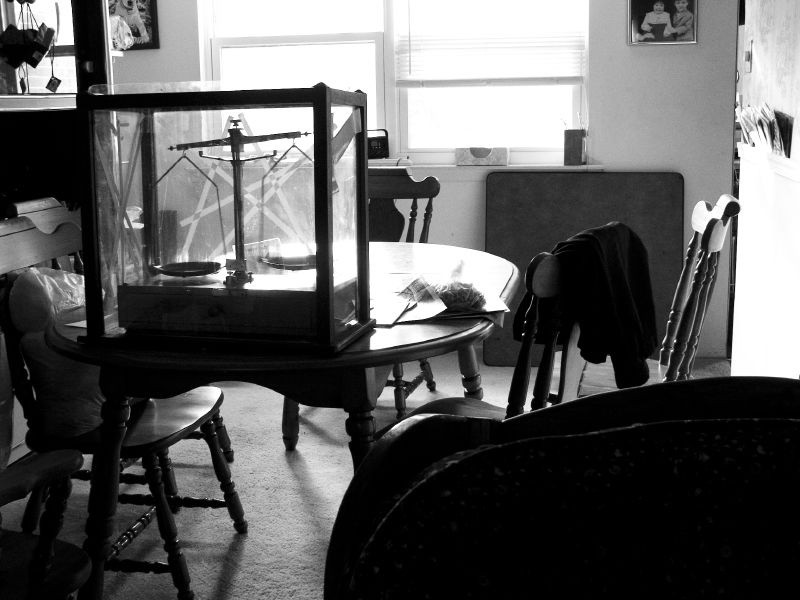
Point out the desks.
[45,242,521,600]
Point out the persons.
[635,0,694,43]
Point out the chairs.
[577,194,741,397]
[283,166,440,451]
[3,266,248,600]
[373,222,625,439]
[52,252,234,513]
[0,332,92,600]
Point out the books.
[370,272,511,328]
[738,102,786,159]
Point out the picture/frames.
[628,0,698,46]
[108,0,160,51]
